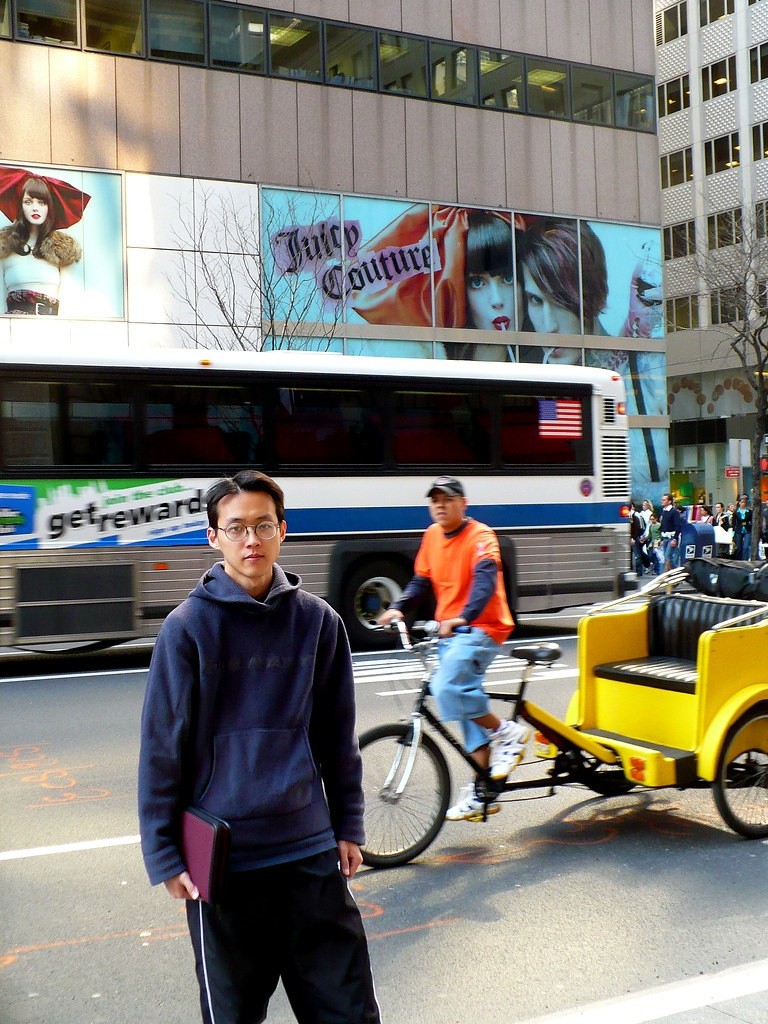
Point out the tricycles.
[334,561,768,867]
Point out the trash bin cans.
[680,523,715,566]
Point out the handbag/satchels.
[714,516,733,543]
[729,542,735,555]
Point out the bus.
[0,350,631,663]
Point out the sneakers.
[488,721,531,780]
[444,783,500,820]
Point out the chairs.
[594,591,768,694]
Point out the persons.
[378,476,529,821]
[631,493,768,577]
[456,213,664,365]
[138,468,384,1024]
[519,217,616,370]
[0,177,82,316]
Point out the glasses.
[218,521,280,542]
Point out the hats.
[427,476,464,497]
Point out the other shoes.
[652,570,656,575]
[645,562,653,574]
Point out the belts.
[8,303,57,315]
